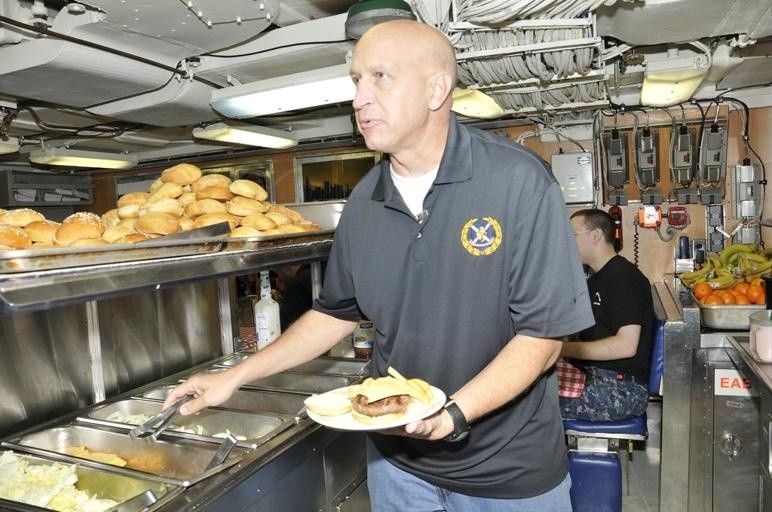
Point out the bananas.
[679,243,772,287]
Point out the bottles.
[352,319,374,359]
[253,270,282,351]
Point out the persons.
[545,209,656,423]
[162,18,596,512]
[280,261,329,336]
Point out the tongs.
[204,435,236,472]
[131,393,193,446]
[136,221,235,244]
[107,488,158,512]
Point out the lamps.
[448,88,507,121]
[206,60,356,119]
[24,146,142,171]
[190,120,300,151]
[637,63,707,110]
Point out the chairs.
[559,317,665,459]
[567,450,624,512]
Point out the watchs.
[442,396,471,444]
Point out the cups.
[748,311,771,362]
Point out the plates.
[302,380,446,434]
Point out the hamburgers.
[347,380,429,423]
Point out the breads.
[0,163,321,254]
[303,392,352,416]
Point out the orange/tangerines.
[691,278,766,306]
